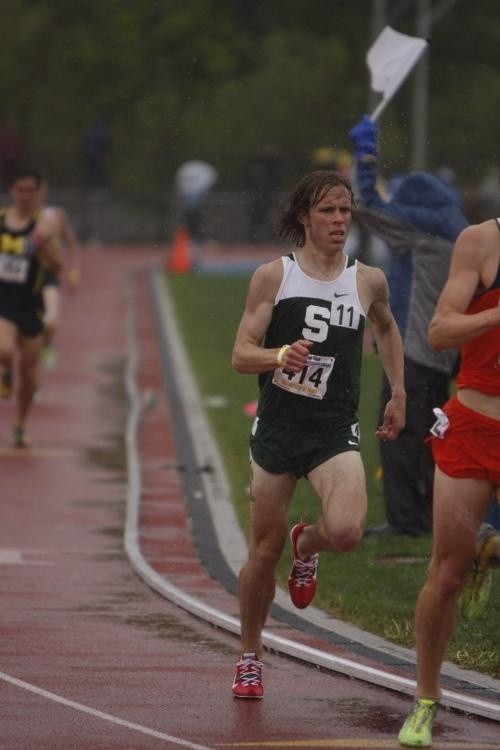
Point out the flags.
[366,24,429,97]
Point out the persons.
[1,117,20,190]
[347,111,473,535]
[231,169,407,700]
[87,113,112,181]
[174,147,216,244]
[0,166,83,447]
[395,207,500,747]
[249,155,280,241]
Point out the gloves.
[348,114,378,157]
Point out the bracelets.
[277,345,290,366]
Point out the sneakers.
[232,652,265,699]
[457,521,497,619]
[288,522,319,609]
[39,343,56,369]
[364,521,432,537]
[398,696,440,747]
[0,375,30,448]
